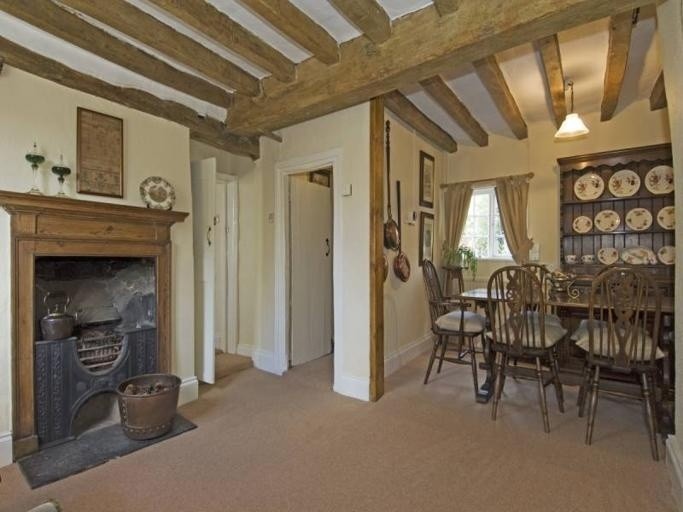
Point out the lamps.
[554,81,590,139]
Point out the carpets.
[19,415,197,489]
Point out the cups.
[565,254,577,265]
[581,253,595,264]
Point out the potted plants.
[443,241,478,278]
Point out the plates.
[656,206,675,231]
[571,214,592,233]
[594,209,620,233]
[624,208,653,232]
[597,246,674,267]
[606,169,641,198]
[642,164,673,195]
[138,176,177,212]
[573,173,604,200]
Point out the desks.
[455,287,675,445]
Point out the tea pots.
[38,291,83,340]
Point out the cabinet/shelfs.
[557,143,674,387]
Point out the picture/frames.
[419,212,434,265]
[419,150,435,208]
[77,106,124,198]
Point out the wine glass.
[24,134,70,200]
